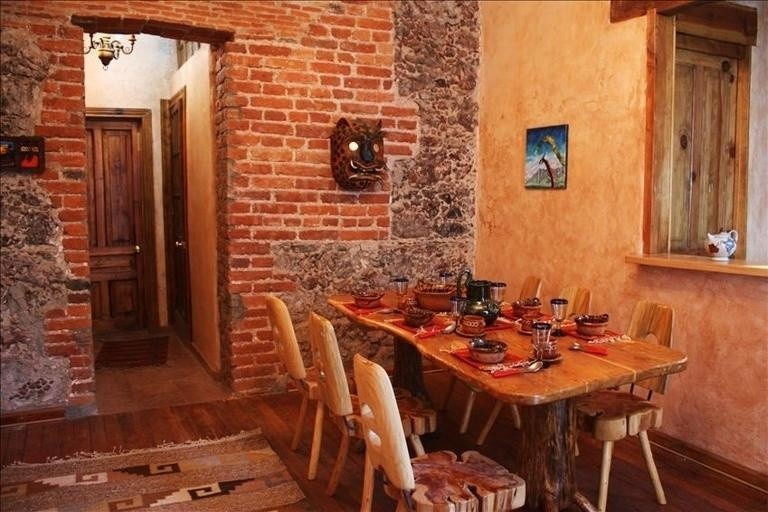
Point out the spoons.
[492,361,542,376]
[568,340,608,356]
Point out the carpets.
[93,336,171,371]
[0,425,305,512]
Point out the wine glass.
[534,322,552,360]
[551,298,568,338]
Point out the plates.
[528,350,564,362]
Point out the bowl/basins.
[469,338,508,363]
[351,266,551,339]
[575,313,610,334]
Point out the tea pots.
[704,229,738,261]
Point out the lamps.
[83,34,137,71]
